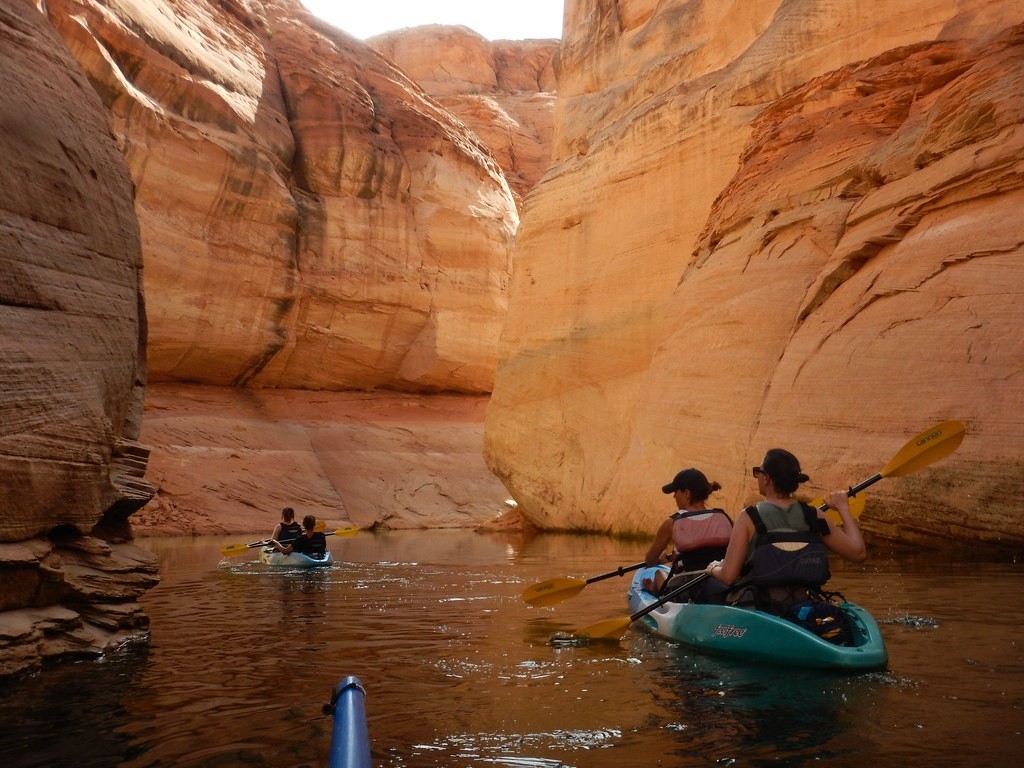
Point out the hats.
[662,468,708,494]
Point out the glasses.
[753,467,765,478]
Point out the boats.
[626,559,889,676]
[258,540,333,568]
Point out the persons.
[641,467,734,602]
[704,448,867,613]
[268,515,327,560]
[261,507,302,553]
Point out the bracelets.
[711,564,718,574]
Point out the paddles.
[220,523,359,556]
[577,419,967,641]
[247,520,327,546]
[520,489,869,609]
